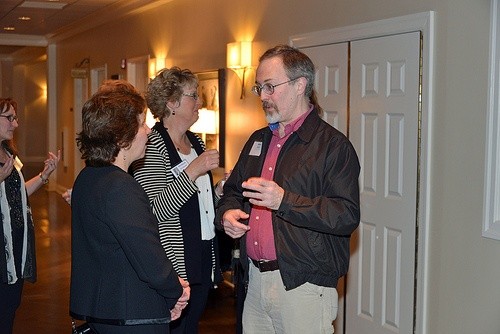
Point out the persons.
[69,80,190,334]
[130,66,233,334]
[213,44,361,334]
[0,97,61,334]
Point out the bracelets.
[39,173,48,184]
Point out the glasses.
[181,91,200,100]
[0,115,19,122]
[251,76,303,96]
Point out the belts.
[252,258,280,272]
